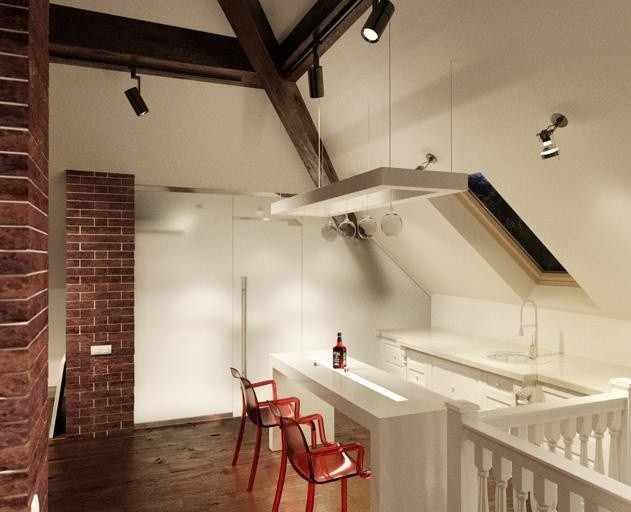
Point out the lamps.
[308,29,325,99]
[360,0,396,45]
[538,112,568,159]
[125,62,149,118]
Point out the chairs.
[229,367,371,511]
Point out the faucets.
[519,299,537,355]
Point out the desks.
[270,350,456,512]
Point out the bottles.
[332,332,347,368]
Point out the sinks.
[486,351,536,366]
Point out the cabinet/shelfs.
[376,337,588,410]
